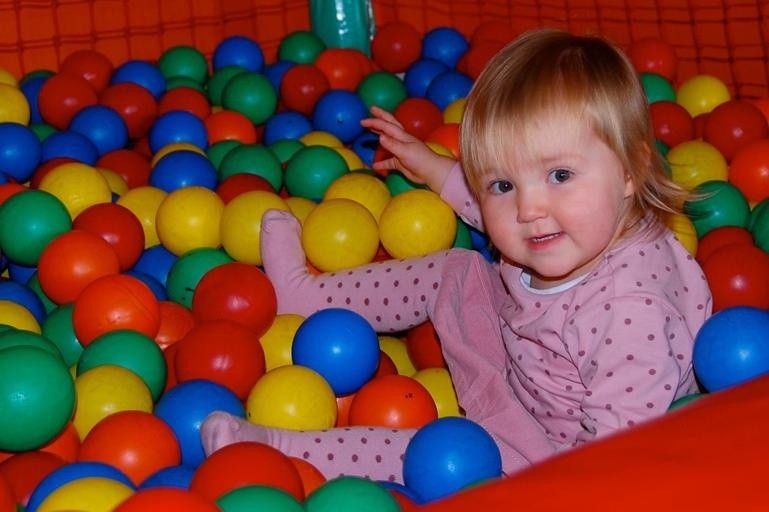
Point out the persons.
[199,27,714,487]
